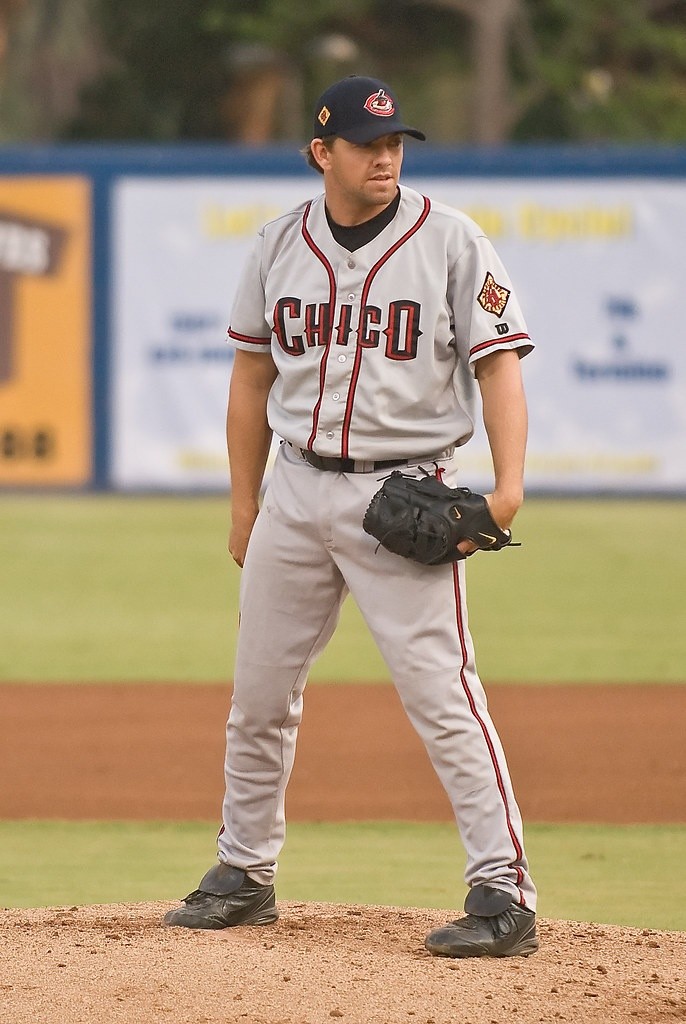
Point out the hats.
[311,73,432,144]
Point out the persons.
[163,75,538,955]
[176,34,302,142]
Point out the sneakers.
[425,883,543,957]
[165,862,280,927]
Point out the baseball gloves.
[360,458,524,567]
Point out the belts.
[292,448,408,471]
